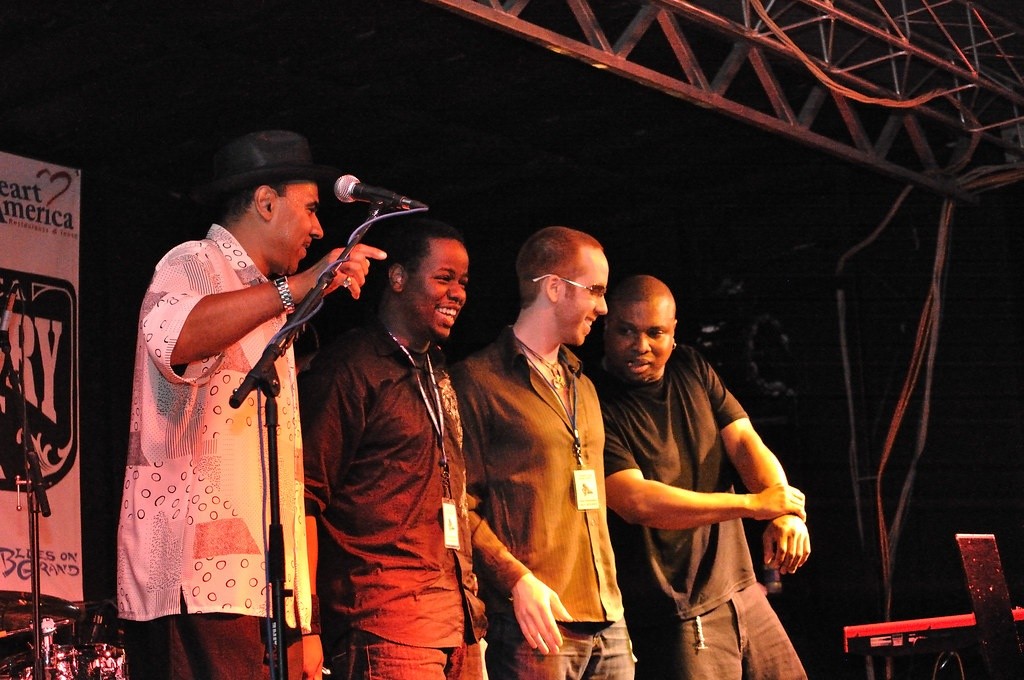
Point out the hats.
[193,128,344,207]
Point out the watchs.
[273,274,296,315]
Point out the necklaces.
[518,337,566,389]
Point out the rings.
[343,275,352,288]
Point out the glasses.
[532,275,607,298]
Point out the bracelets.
[294,594,322,638]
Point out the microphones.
[0,283,18,337]
[334,174,427,210]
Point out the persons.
[115,127,387,680]
[583,274,812,679]
[292,223,490,680]
[446,223,640,680]
